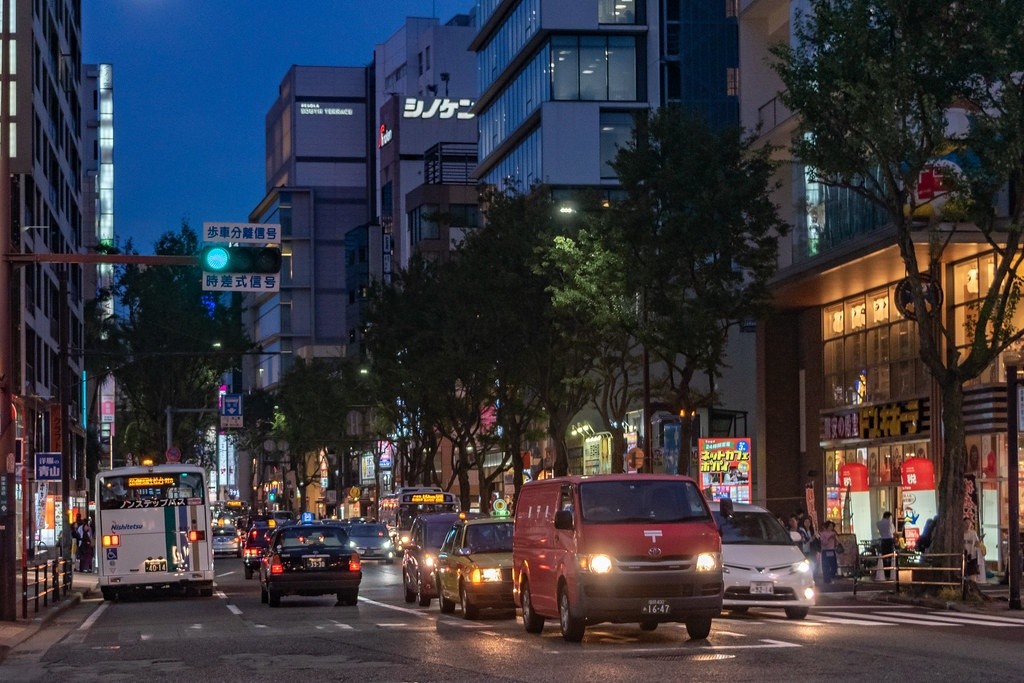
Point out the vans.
[513,473,724,641]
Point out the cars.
[211,509,394,579]
[434,517,518,620]
[402,513,488,606]
[259,513,363,607]
[707,501,817,619]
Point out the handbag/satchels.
[826,551,834,557]
[980,540,986,556]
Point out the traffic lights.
[201,246,282,273]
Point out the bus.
[378,487,462,557]
[95,462,214,601]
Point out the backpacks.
[915,537,931,551]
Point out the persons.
[588,496,617,516]
[964,517,981,592]
[777,515,841,585]
[58,514,95,573]
[921,515,939,560]
[968,435,996,489]
[877,512,895,580]
[826,447,925,484]
[505,495,512,510]
[490,494,497,509]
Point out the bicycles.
[860,540,880,576]
